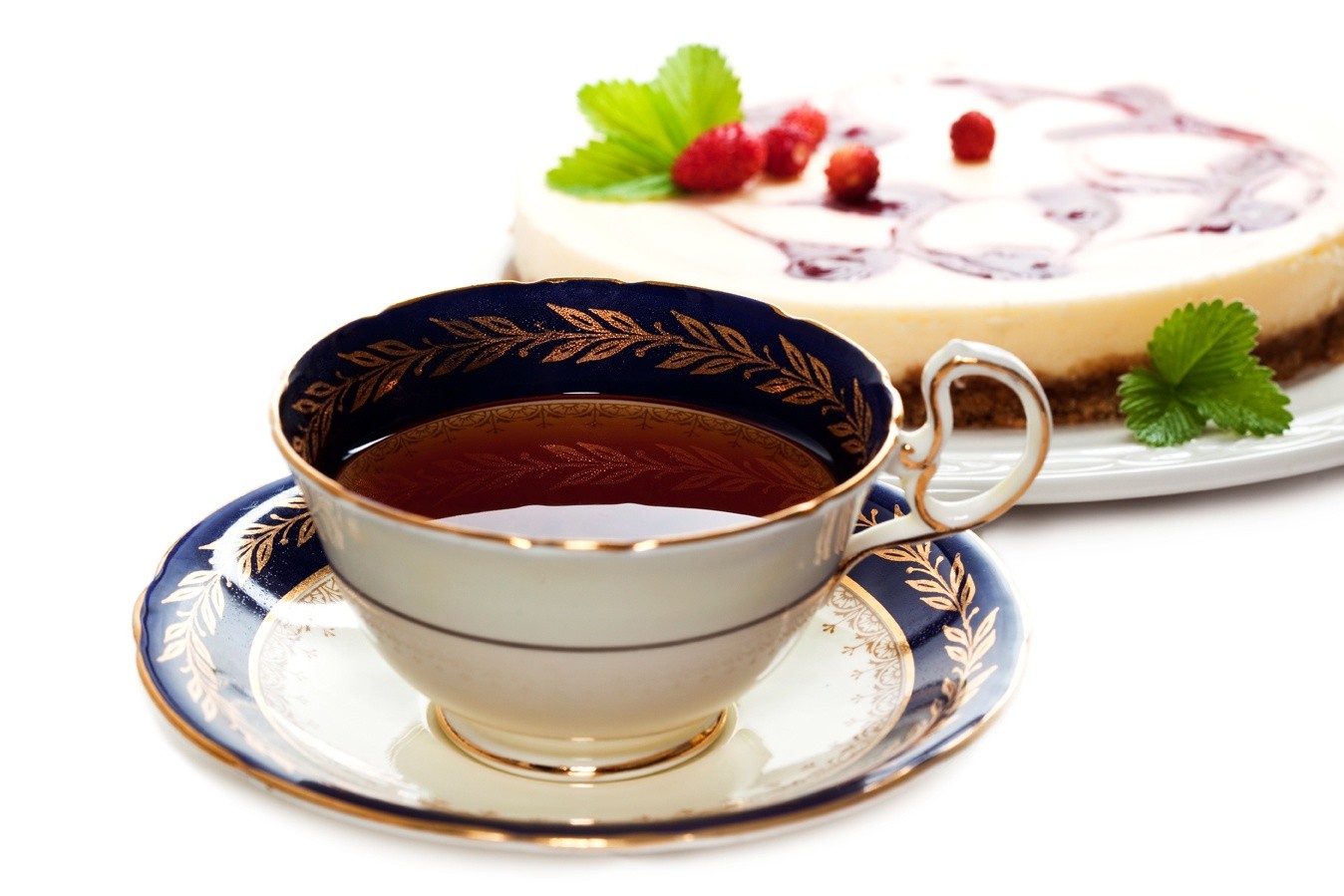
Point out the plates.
[131,475,1027,858]
[879,358,1344,505]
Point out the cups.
[269,275,1054,785]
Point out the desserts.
[509,43,1344,446]
